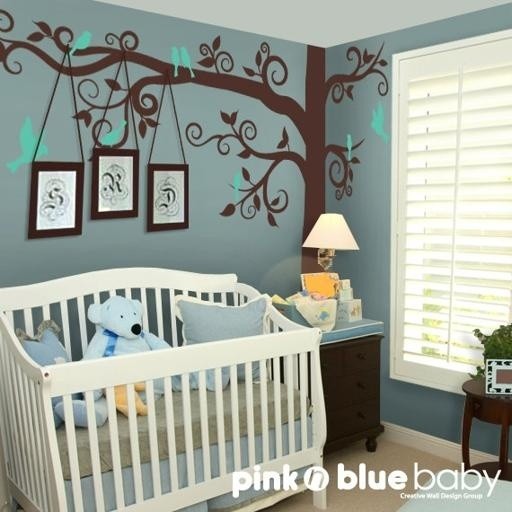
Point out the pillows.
[173,293,272,384]
[14,321,82,430]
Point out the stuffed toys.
[56,294,231,428]
[103,381,152,418]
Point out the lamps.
[301,212,360,270]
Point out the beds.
[0,267,327,512]
[461,379,512,482]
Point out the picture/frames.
[29,162,86,238]
[485,358,512,396]
[146,162,189,232]
[91,147,140,221]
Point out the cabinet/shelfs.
[319,333,386,456]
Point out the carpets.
[396,469,512,512]
